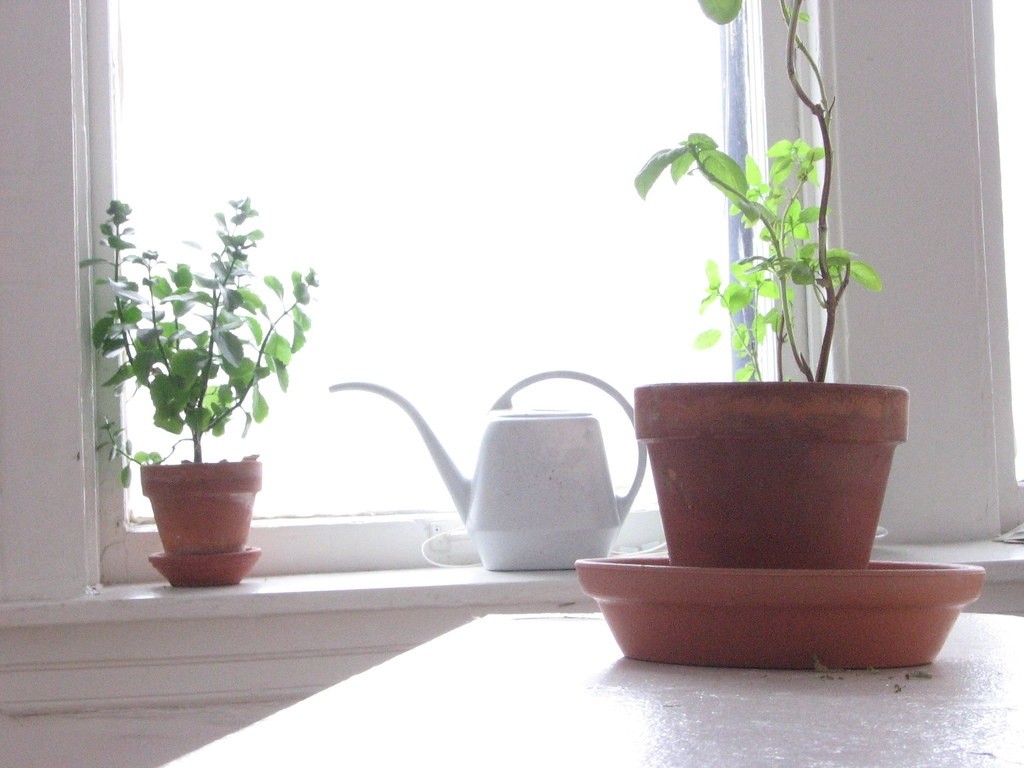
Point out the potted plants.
[631,0,908,568]
[71,195,319,551]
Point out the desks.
[159,612,1024,768]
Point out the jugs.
[328,371,647,571]
